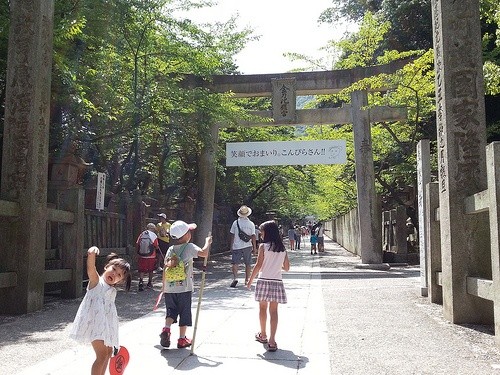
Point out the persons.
[228,204,257,288]
[135,222,160,291]
[158,219,213,352]
[250,219,327,255]
[67,245,134,375]
[245,219,292,354]
[155,213,172,272]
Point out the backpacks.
[164,243,189,284]
[137,231,156,256]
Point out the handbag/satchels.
[238,230,251,242]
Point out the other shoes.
[255,332,269,343]
[230,279,239,288]
[147,281,154,288]
[245,278,249,285]
[160,327,172,348]
[177,335,194,349]
[267,341,277,351]
[291,248,300,250]
[310,251,317,255]
[138,280,144,291]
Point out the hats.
[169,220,197,240]
[147,223,159,232]
[237,205,253,218]
[157,212,166,219]
